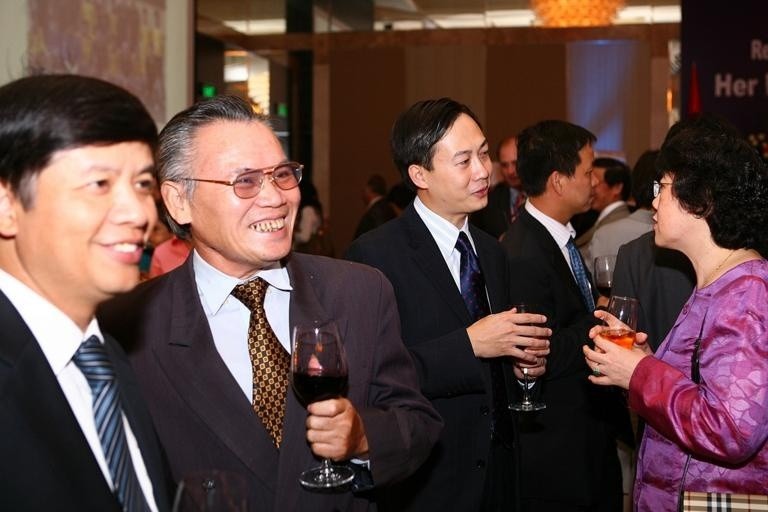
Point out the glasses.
[172,161,304,199]
[652,180,672,196]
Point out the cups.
[592,252,617,297]
[597,293,646,358]
[170,470,249,511]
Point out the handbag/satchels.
[677,492,768,512]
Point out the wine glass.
[504,302,551,414]
[288,313,360,491]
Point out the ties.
[455,232,495,444]
[73,334,152,511]
[232,278,292,450]
[566,237,595,315]
